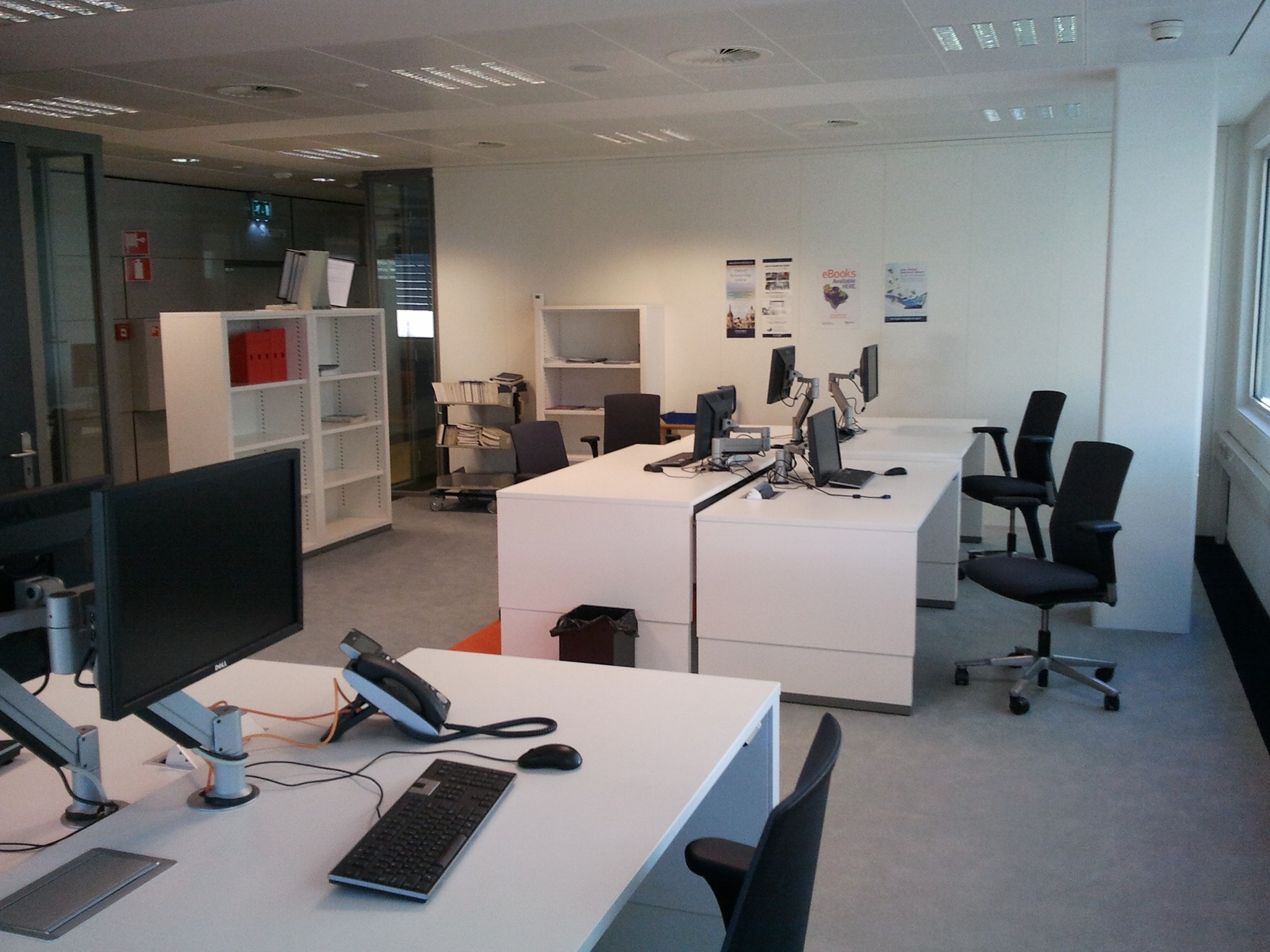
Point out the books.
[320,365,340,376]
[431,372,524,407]
[321,414,369,426]
[436,424,511,448]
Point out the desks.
[0,417,994,952]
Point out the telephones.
[338,627,453,736]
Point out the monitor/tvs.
[692,384,771,462]
[0,473,130,683]
[88,447,305,721]
[859,342,880,403]
[767,345,795,404]
[772,406,844,489]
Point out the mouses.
[644,463,664,473]
[885,466,908,477]
[514,741,586,772]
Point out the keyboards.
[652,451,692,466]
[324,755,519,907]
[827,467,874,490]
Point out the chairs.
[580,393,680,458]
[961,388,1068,559]
[686,713,841,952]
[511,421,570,478]
[952,440,1135,714]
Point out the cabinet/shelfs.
[429,381,526,514]
[158,308,393,554]
[539,303,640,462]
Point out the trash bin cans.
[559,604,636,668]
